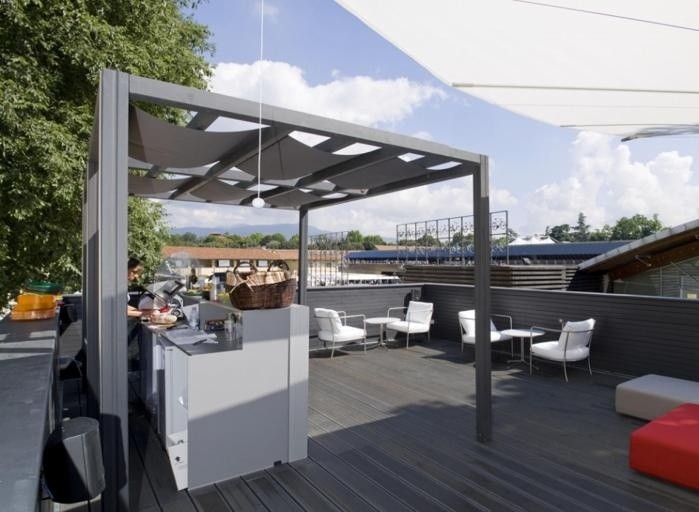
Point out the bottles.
[190,307,199,329]
[224,312,242,341]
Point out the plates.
[157,323,175,328]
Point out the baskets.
[224,260,296,310]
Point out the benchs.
[630,403,699,489]
[615,374,699,421]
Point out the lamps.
[251,103,265,207]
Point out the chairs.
[386,301,434,348]
[312,307,366,358]
[530,318,595,382]
[458,309,513,357]
[54,358,82,416]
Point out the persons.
[125,257,159,321]
